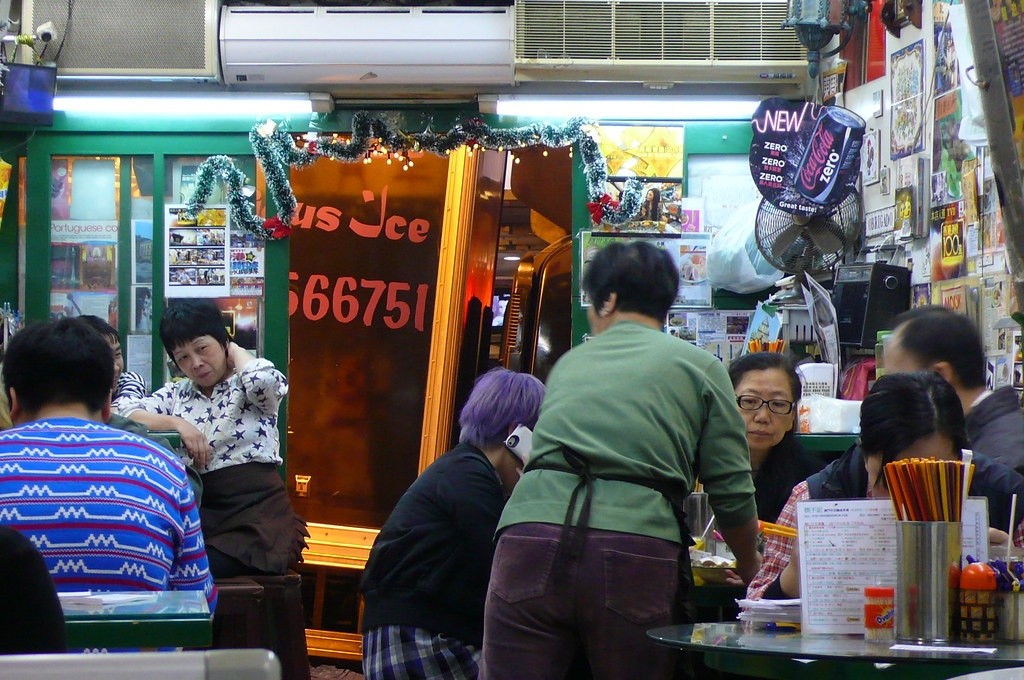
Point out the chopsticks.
[883,456,976,522]
[747,337,786,354]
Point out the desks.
[645,621,1024,680]
[55,590,213,650]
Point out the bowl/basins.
[690,558,738,584]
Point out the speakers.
[831,263,910,350]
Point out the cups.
[893,520,964,646]
[682,492,709,539]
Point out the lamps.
[478,93,778,120]
[50,91,335,117]
[781,0,873,79]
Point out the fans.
[755,187,864,304]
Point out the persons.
[741,371,1024,626]
[727,352,826,524]
[0,317,217,655]
[478,243,762,680]
[641,188,667,221]
[361,368,546,680]
[115,298,310,580]
[77,315,145,404]
[882,304,1024,474]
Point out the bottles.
[864,586,897,644]
[958,562,997,645]
[874,330,895,380]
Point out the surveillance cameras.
[37,22,58,43]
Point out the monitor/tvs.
[0,62,58,127]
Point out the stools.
[212,566,311,680]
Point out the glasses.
[735,395,795,415]
